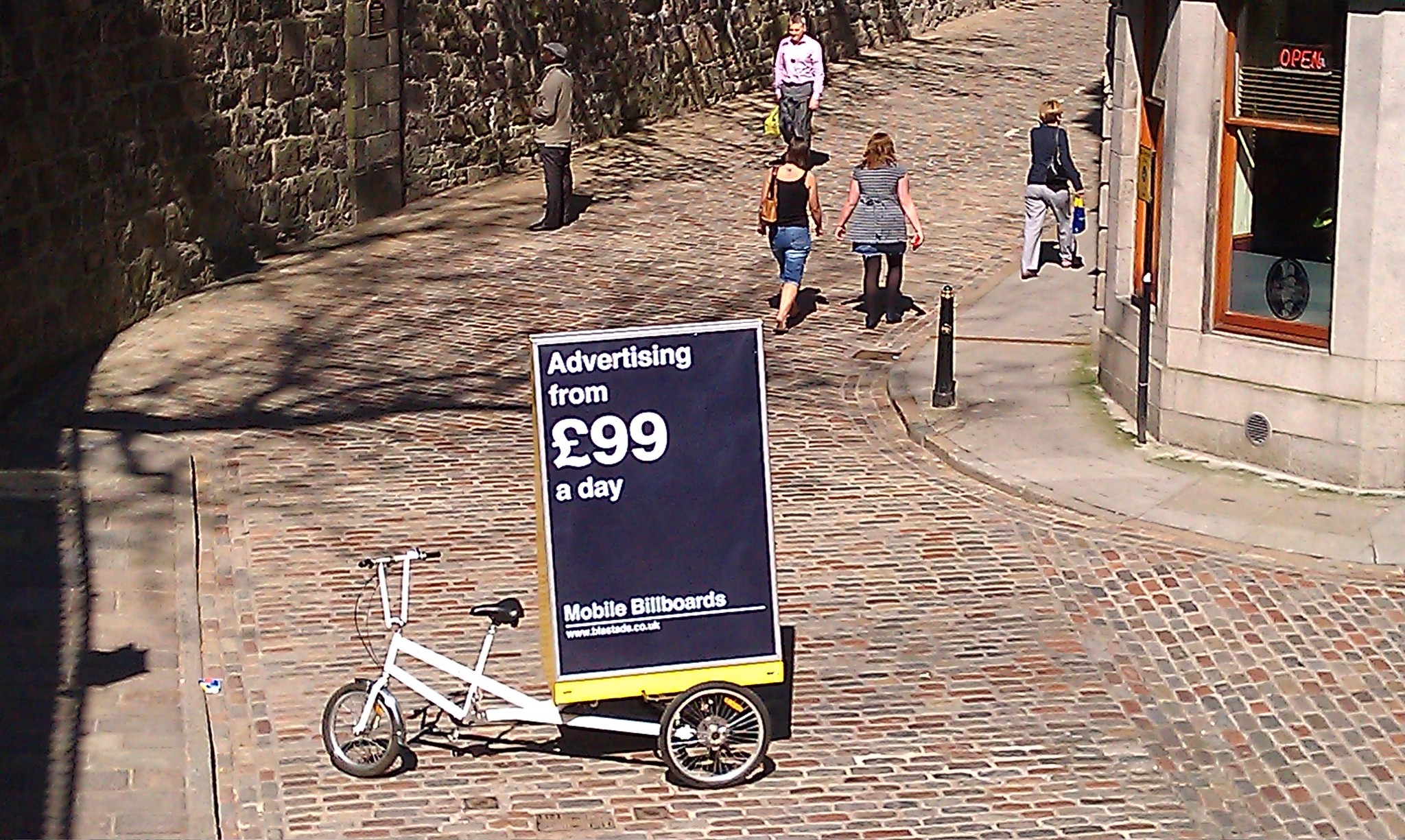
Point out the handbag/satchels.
[1046,130,1073,181]
[759,164,780,225]
[764,103,781,137]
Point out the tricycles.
[319,543,797,789]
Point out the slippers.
[773,326,788,333]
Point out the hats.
[543,41,569,61]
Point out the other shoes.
[866,316,876,329]
[1021,269,1037,278]
[529,221,550,231]
[1062,260,1075,268]
[885,313,901,323]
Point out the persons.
[835,133,925,330]
[774,13,824,148]
[757,136,822,333]
[1020,100,1085,278]
[523,42,575,231]
